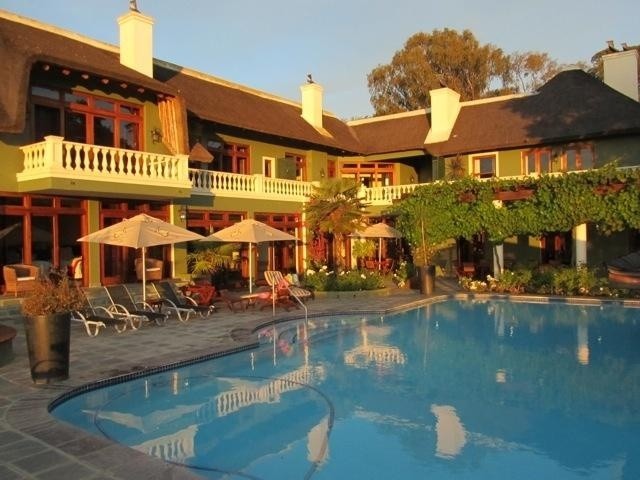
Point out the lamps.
[176,205,187,221]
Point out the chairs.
[360,255,395,273]
[264,270,312,307]
[70,281,215,337]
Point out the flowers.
[301,264,386,291]
[393,260,417,288]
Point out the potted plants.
[17,268,83,385]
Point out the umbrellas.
[197,219,303,302]
[348,221,407,274]
[76,213,207,311]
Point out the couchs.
[132,257,165,282]
[2,262,42,298]
